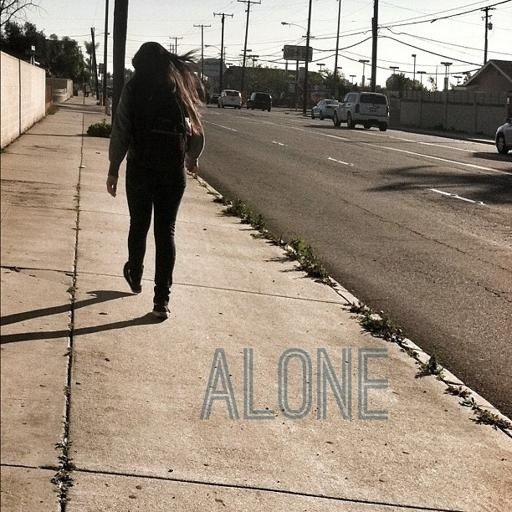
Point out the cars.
[210,94,221,104]
[495,116,512,156]
[311,98,339,120]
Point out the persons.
[106,41,206,318]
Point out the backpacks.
[125,76,186,174]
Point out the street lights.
[204,45,226,64]
[281,22,461,91]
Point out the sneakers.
[123,260,142,293]
[151,304,171,318]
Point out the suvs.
[246,92,272,112]
[218,89,242,109]
[333,90,391,131]
